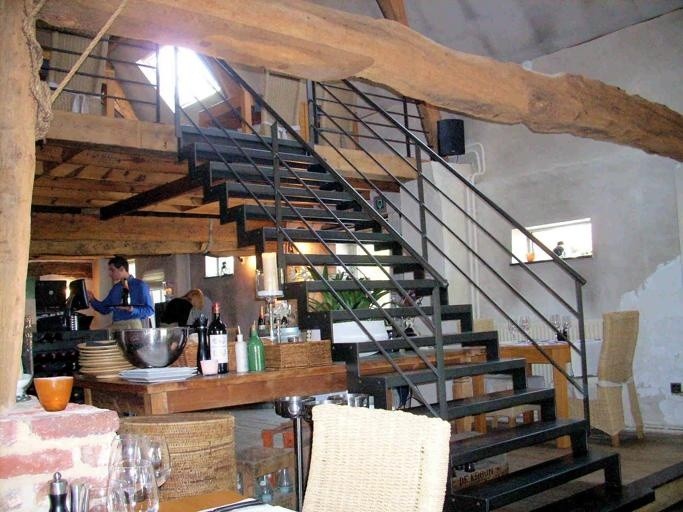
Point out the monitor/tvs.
[69,279,89,311]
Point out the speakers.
[437,119,465,156]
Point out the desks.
[72,363,347,415]
[497,339,601,449]
[199,92,261,133]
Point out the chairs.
[568,310,644,448]
[237,70,304,140]
[302,404,451,512]
[46,34,109,114]
[317,84,356,149]
[457,319,544,429]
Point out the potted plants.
[292,263,404,358]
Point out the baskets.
[177,342,236,373]
[262,339,332,371]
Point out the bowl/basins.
[115,326,189,369]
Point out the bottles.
[122,278,131,304]
[44,471,70,512]
[236,465,292,502]
[197,302,266,375]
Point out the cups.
[107,433,172,512]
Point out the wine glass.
[17,373,33,403]
[508,314,571,343]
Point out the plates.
[76,339,198,382]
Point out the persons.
[154,288,205,327]
[85,256,154,330]
